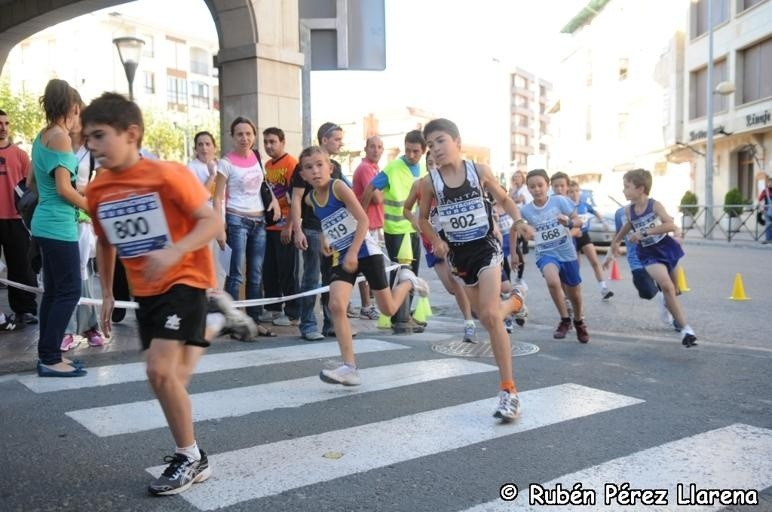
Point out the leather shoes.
[37,357,87,377]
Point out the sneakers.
[208,290,257,343]
[321,367,360,386]
[1,312,39,333]
[399,269,429,297]
[601,287,613,298]
[149,449,209,496]
[554,297,589,343]
[464,323,479,343]
[360,305,380,319]
[392,310,427,333]
[259,305,359,341]
[60,334,73,351]
[682,330,698,348]
[493,388,520,420]
[501,278,528,332]
[86,328,103,346]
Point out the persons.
[0,80,697,496]
[758,178,772,244]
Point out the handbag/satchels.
[261,181,282,224]
[17,188,39,230]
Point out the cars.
[542,188,632,246]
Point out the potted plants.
[722,188,746,230]
[680,190,699,229]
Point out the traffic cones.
[726,272,751,301]
[608,258,624,281]
[676,264,691,293]
[375,288,434,329]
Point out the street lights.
[112,26,146,102]
[703,0,735,241]
